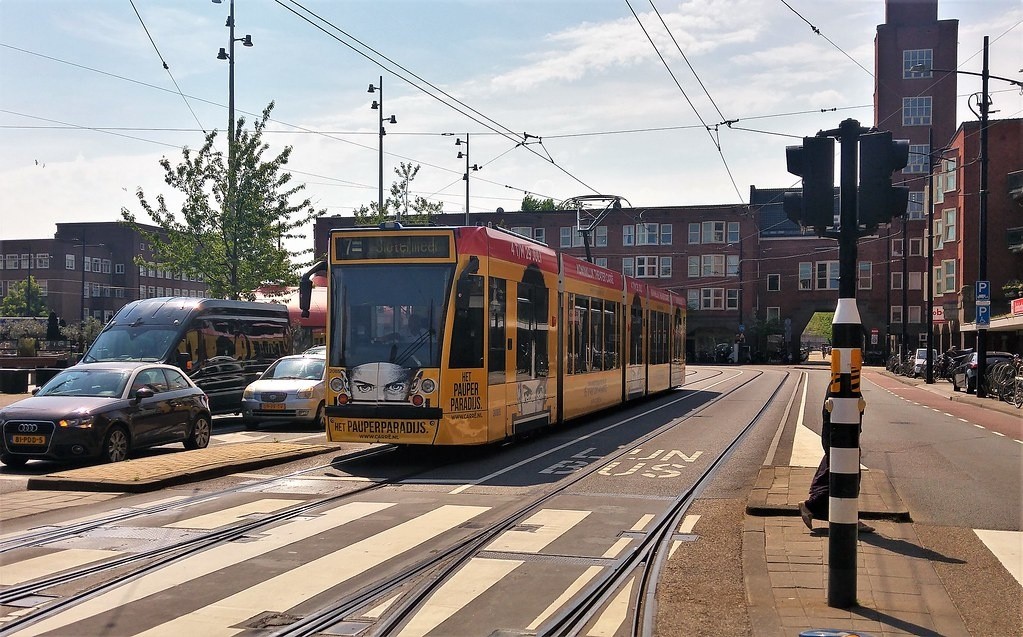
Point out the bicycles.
[889,351,1023,410]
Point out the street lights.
[908,34,1022,390]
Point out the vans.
[912,348,938,378]
[71,296,293,416]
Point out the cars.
[951,352,1015,395]
[240,353,326,429]
[821,343,832,356]
[799,346,810,361]
[0,363,214,465]
[299,344,326,360]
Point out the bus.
[299,194,688,449]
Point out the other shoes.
[798,501,813,530]
[857,521,875,531]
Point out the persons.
[629,365,643,389]
[798,353,875,533]
[339,343,424,403]
[517,381,547,413]
[394,315,438,345]
[822,344,827,360]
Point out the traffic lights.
[782,135,835,229]
[859,130,911,228]
